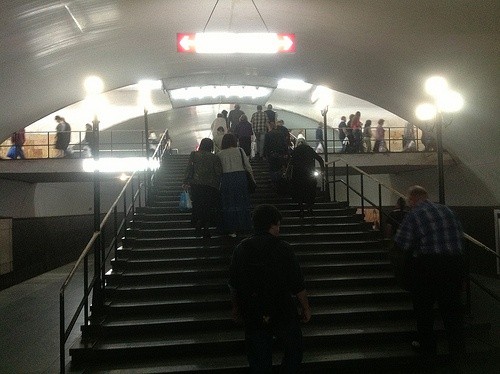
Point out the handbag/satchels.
[178,188,192,212]
[239,148,257,193]
[7,146,18,159]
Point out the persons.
[383,198,411,281]
[340,110,388,153]
[315,122,325,149]
[183,139,222,238]
[85,124,94,157]
[404,122,416,152]
[11,128,26,159]
[216,133,256,237]
[145,131,171,156]
[55,116,71,157]
[287,138,324,218]
[210,104,290,176]
[390,185,470,374]
[229,206,311,374]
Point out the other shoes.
[227,232,237,238]
[411,341,422,353]
